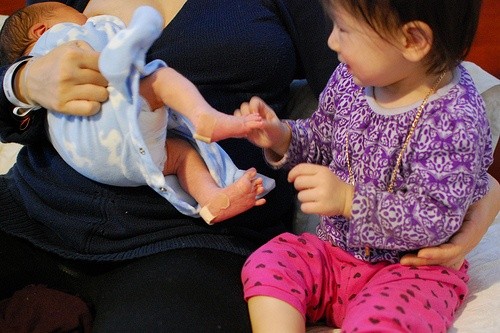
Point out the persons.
[233,1,493,333]
[1,1,499,332]
[1,4,268,224]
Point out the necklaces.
[348,66,448,256]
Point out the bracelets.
[4,57,41,115]
